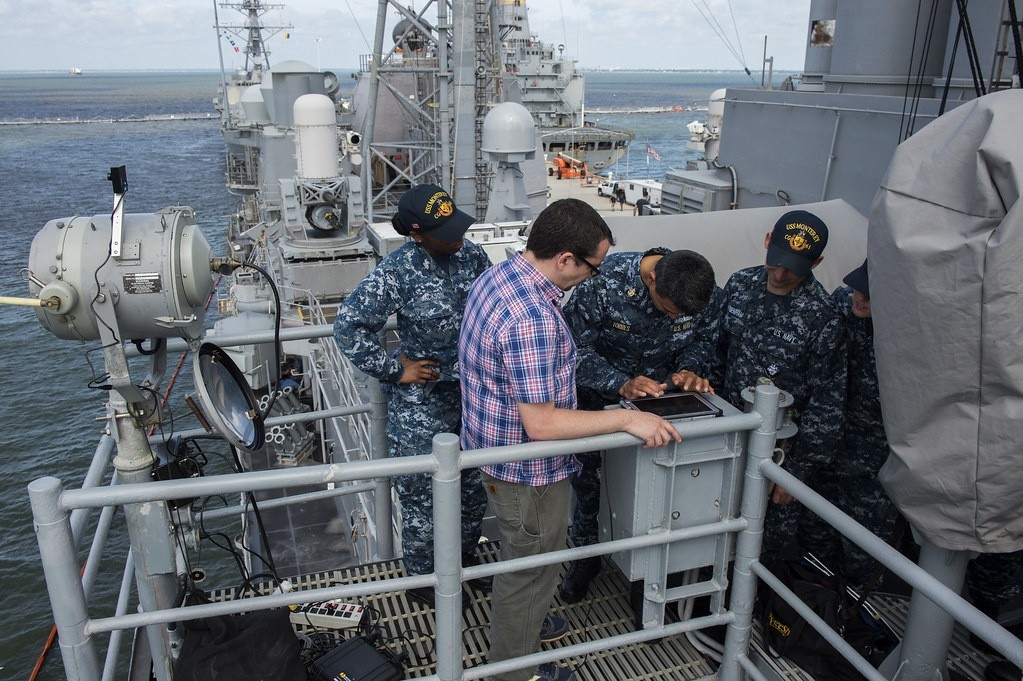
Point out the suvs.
[598,181,618,198]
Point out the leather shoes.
[462,557,494,593]
[405,586,471,611]
[631,596,664,644]
[560,555,601,604]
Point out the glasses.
[561,250,601,278]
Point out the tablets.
[625,391,719,419]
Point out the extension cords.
[289,601,364,631]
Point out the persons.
[561,247,721,603]
[700,210,890,614]
[608,191,618,211]
[619,190,626,211]
[635,192,653,215]
[387,156,396,184]
[334,184,493,608]
[459,199,684,681]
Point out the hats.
[398,184,477,244]
[842,257,870,299]
[766,210,829,277]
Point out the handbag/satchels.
[761,580,896,681]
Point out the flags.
[647,146,659,160]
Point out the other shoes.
[539,616,568,642]
[528,664,576,681]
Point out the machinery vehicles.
[548,151,586,180]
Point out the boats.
[499,0,633,175]
[212,0,298,196]
